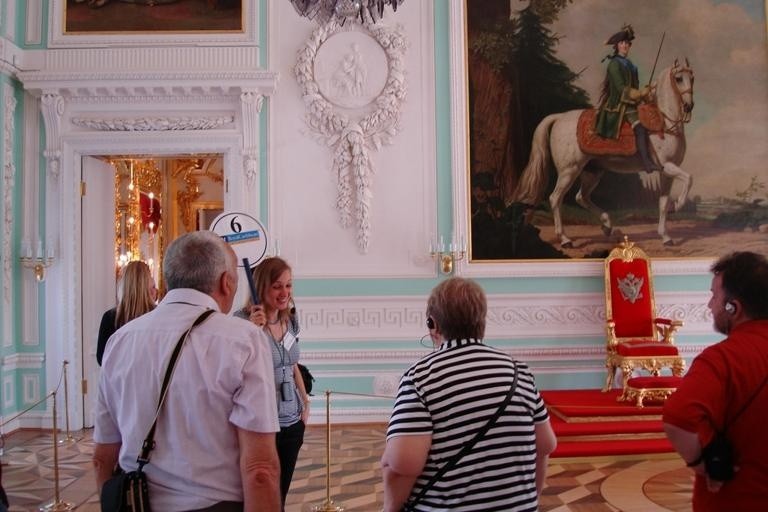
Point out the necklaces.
[265,317,280,325]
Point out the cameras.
[281,381,293,401]
[702,435,740,483]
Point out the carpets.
[531,387,679,458]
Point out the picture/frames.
[47,1,261,48]
[450,0,767,278]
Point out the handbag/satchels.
[298,362,316,396]
[101,464,149,512]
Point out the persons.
[379,277,558,511]
[91,229,286,512]
[660,249,767,511]
[148,279,158,301]
[94,257,158,367]
[349,43,369,98]
[230,255,310,511]
[594,27,665,176]
[332,54,354,98]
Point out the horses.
[509,55,696,250]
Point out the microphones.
[290,298,296,314]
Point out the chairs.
[601,235,686,409]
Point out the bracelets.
[684,454,704,469]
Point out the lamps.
[18,240,60,283]
[428,233,468,274]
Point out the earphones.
[426,317,435,329]
[726,303,736,315]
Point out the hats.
[605,26,635,45]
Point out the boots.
[631,121,666,174]
